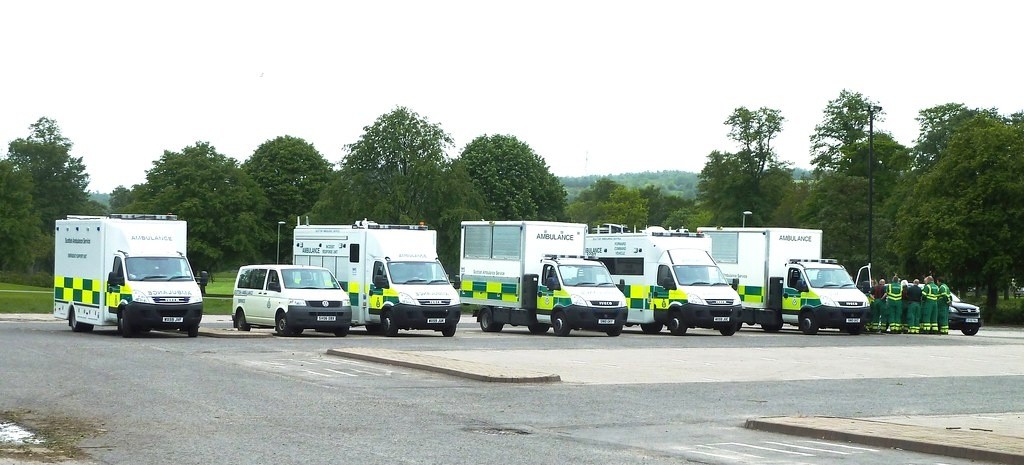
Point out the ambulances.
[53,213,208,338]
[292,216,462,337]
[457,219,629,338]
[583,223,743,336]
[695,226,870,335]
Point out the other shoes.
[865,328,948,335]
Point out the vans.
[233,264,352,338]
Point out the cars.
[883,284,982,336]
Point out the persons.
[865,275,952,334]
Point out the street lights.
[742,210,753,227]
[276,221,287,262]
[866,105,884,283]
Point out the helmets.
[879,279,885,285]
[901,280,908,287]
